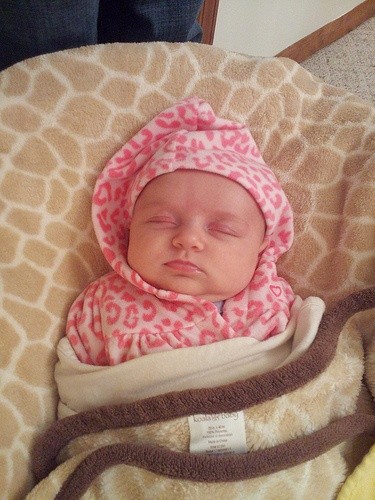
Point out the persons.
[55,94,330,499]
[1,0,205,77]
